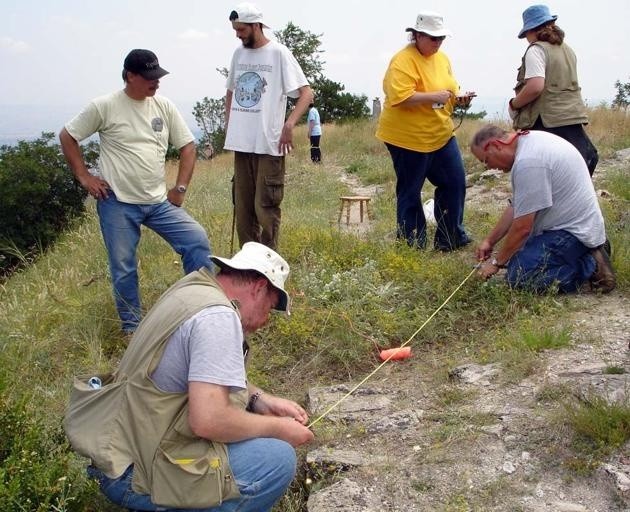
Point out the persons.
[62,241,315,511]
[508,4,599,177]
[59,47,215,332]
[204,141,213,159]
[307,100,322,164]
[223,7,314,250]
[375,16,476,252]
[470,124,617,294]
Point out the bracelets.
[247,391,263,413]
[509,98,514,108]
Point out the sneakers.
[593,247,617,293]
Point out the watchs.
[492,257,504,270]
[175,186,187,193]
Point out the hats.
[122,49,169,80]
[517,4,559,38]
[405,13,452,39]
[227,3,271,30]
[207,241,291,314]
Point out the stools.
[338,196,373,227]
